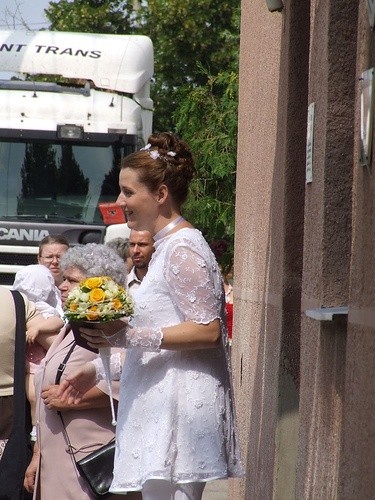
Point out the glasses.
[39,254,63,263]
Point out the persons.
[208,239,235,349]
[36,235,73,288]
[23,244,128,500]
[13,265,63,445]
[126,227,157,296]
[55,133,243,500]
[1,285,62,500]
[106,236,134,275]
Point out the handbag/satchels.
[0,428,35,500]
[77,437,116,500]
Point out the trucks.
[0,29,154,287]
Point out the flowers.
[63,275,135,322]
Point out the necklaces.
[152,216,184,241]
